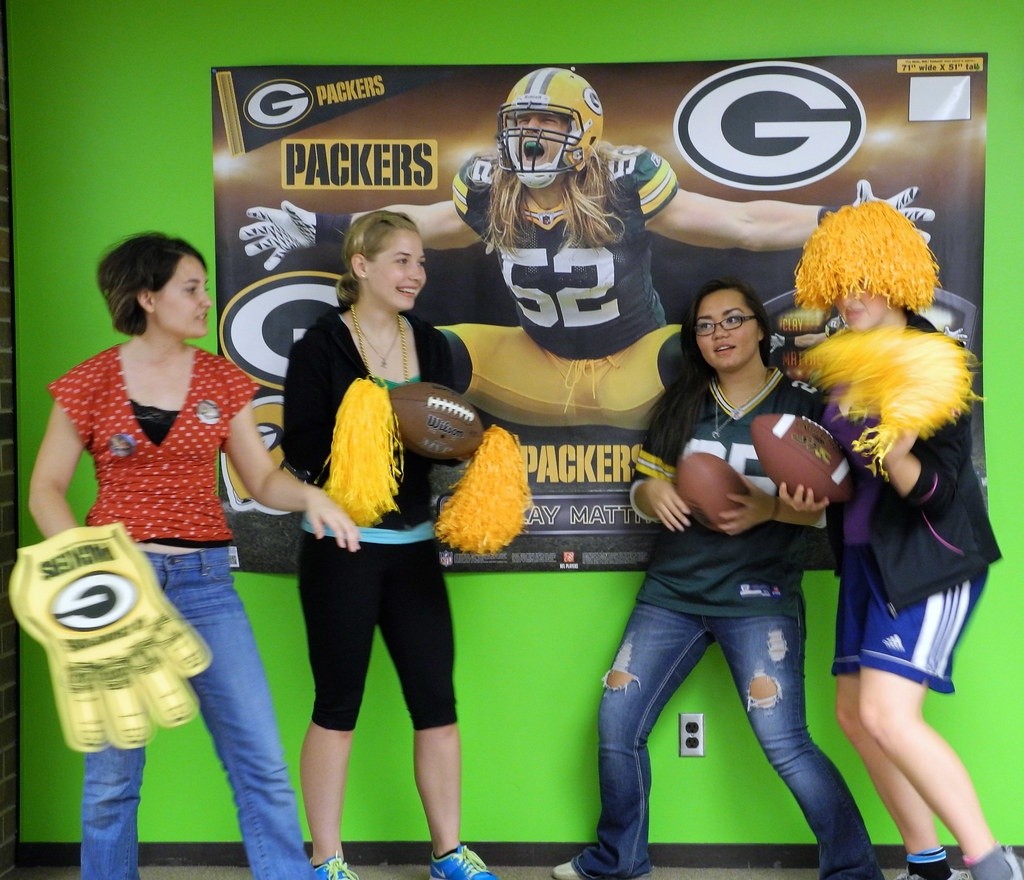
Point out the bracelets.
[771,494,780,519]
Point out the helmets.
[501,68,605,172]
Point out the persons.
[552,278,885,880]
[795,200,1023,880]
[238,67,935,429]
[28,232,362,880]
[285,211,500,880]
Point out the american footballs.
[677,453,751,532]
[750,413,852,503]
[388,381,484,459]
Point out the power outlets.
[679,713,704,758]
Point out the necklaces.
[351,304,410,384]
[358,321,401,369]
[712,373,772,439]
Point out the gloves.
[7,520,214,751]
[237,200,349,271]
[817,179,937,245]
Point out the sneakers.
[429,844,500,880]
[310,851,360,880]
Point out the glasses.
[694,315,756,336]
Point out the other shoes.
[890,865,971,880]
[1003,843,1024,880]
[551,859,586,880]
